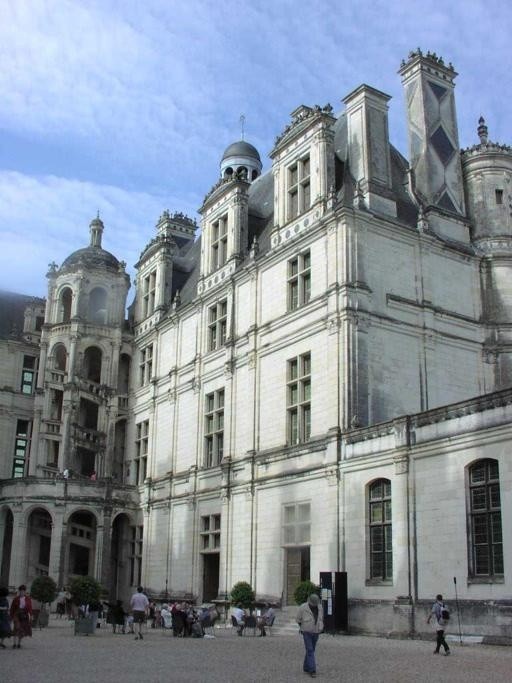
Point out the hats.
[308,594,319,605]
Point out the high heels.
[0,643,21,648]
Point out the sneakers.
[433,651,451,656]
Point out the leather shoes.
[303,668,317,678]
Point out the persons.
[296,594,324,677]
[427,594,451,656]
[90,471,96,480]
[0,584,34,649]
[63,468,69,478]
[103,584,275,649]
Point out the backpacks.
[437,603,450,626]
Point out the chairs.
[232,612,275,638]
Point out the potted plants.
[69,574,102,635]
[29,576,57,627]
